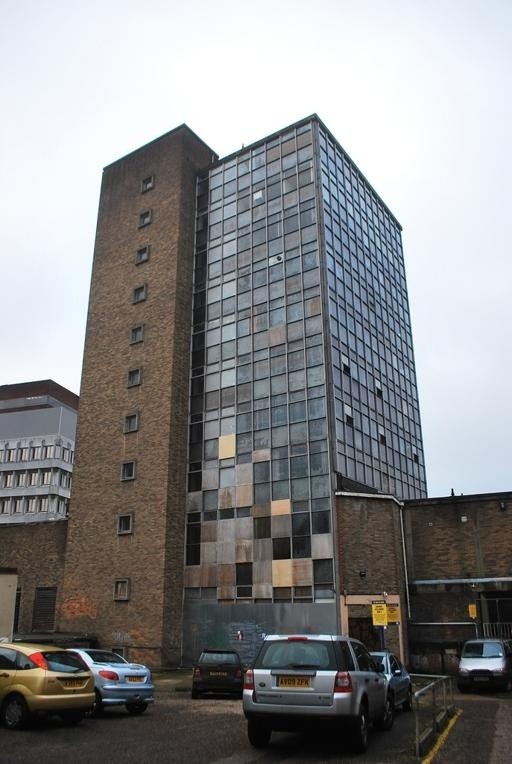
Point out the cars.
[0,642,99,730]
[364,650,414,713]
[190,647,248,701]
[61,647,156,719]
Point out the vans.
[454,637,512,694]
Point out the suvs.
[241,632,399,755]
